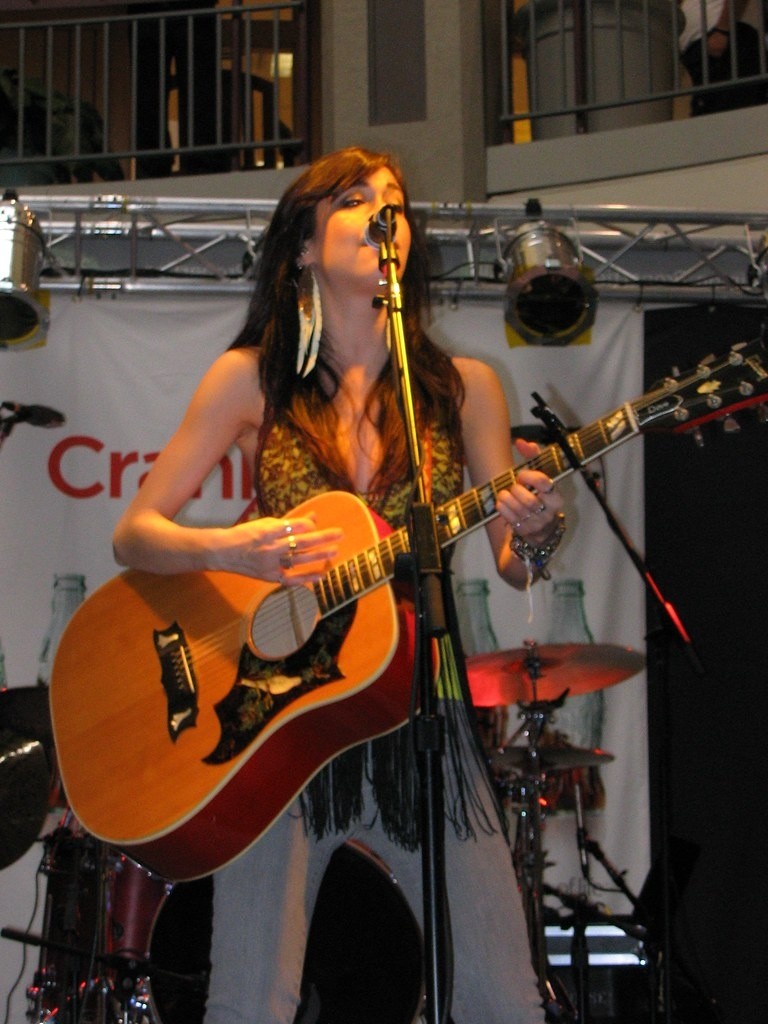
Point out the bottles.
[35,574,87,690]
[455,580,507,750]
[541,579,607,814]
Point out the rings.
[521,511,532,521]
[544,480,556,494]
[511,521,521,528]
[281,552,293,568]
[282,519,294,535]
[277,574,285,586]
[288,536,296,550]
[536,503,545,514]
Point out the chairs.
[135,70,276,174]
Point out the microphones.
[573,784,589,879]
[364,203,397,250]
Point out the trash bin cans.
[517,0,687,146]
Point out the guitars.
[46,339,768,887]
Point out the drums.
[145,836,425,1024]
[25,824,173,1024]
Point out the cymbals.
[464,643,649,706]
[480,728,616,774]
[0,685,63,871]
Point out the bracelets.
[712,27,731,37]
[509,512,566,566]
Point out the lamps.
[499,220,599,348]
[1,188,52,352]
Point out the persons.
[113,149,566,1023]
[676,0,768,118]
